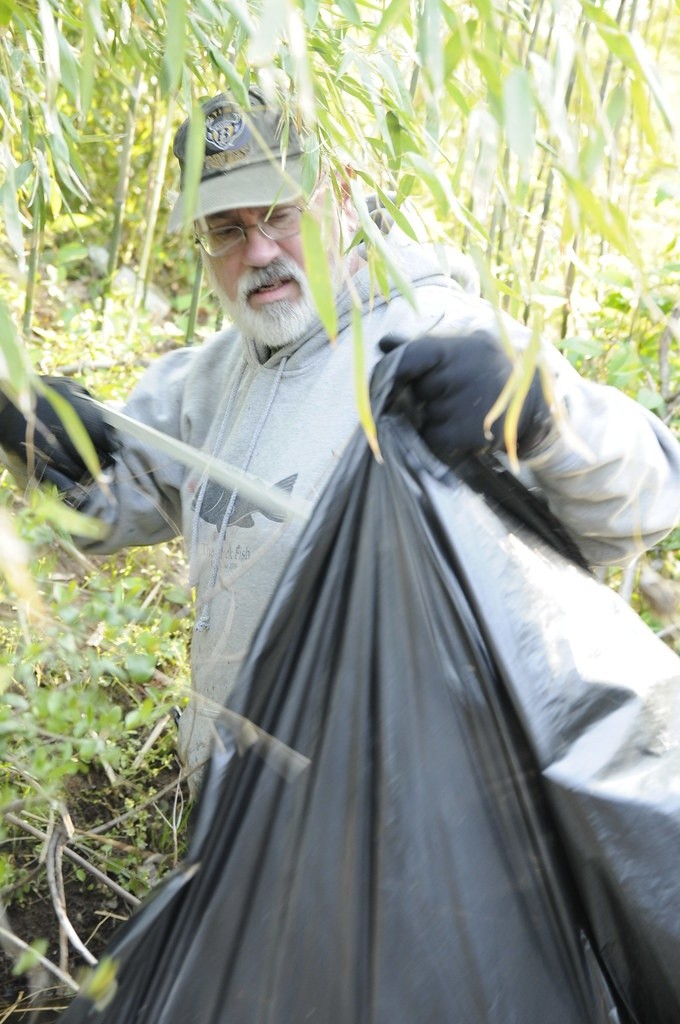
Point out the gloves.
[378,330,543,466]
[0,374,122,508]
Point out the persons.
[0,88,680,838]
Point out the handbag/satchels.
[62,341,636,1024]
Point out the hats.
[166,86,316,234]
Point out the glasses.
[194,202,311,256]
